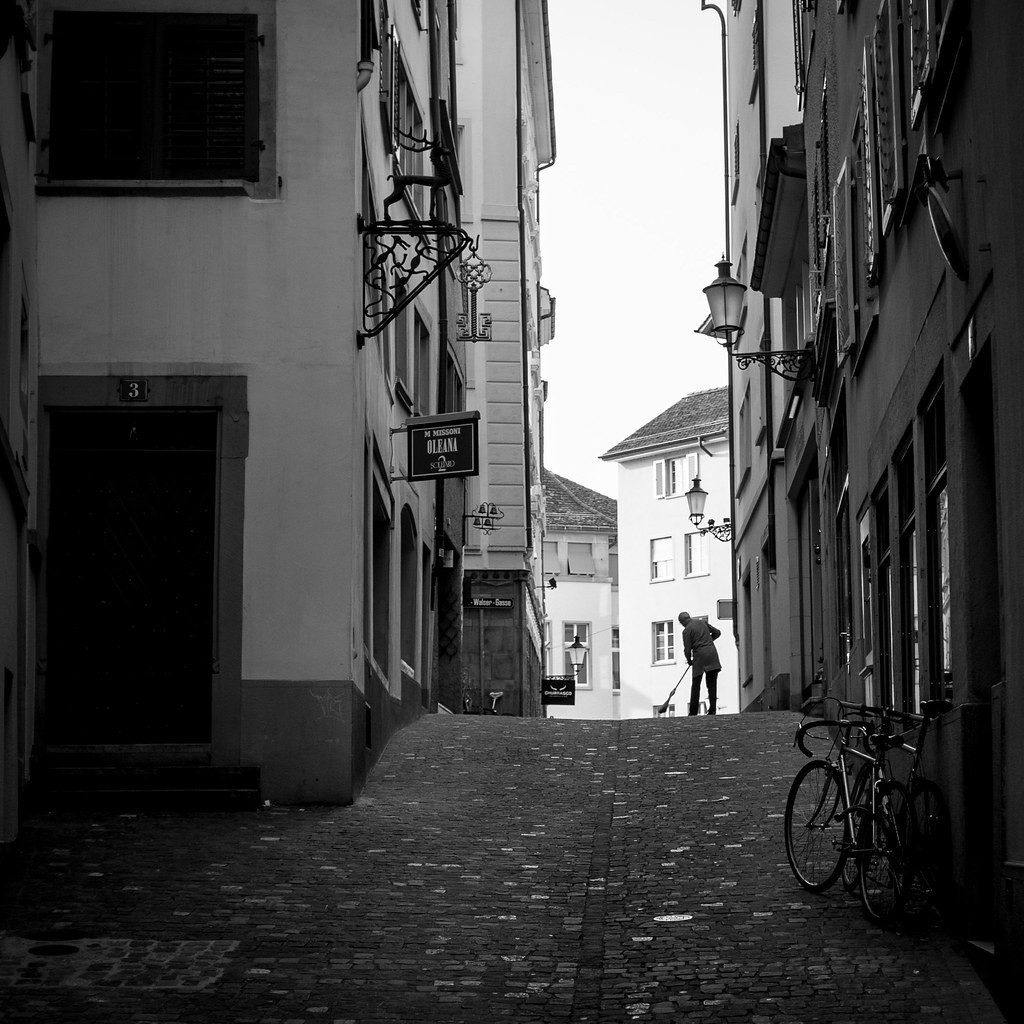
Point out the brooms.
[657,664,691,714]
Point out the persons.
[678,612,721,716]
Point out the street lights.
[702,253,748,643]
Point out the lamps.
[535,578,556,589]
[546,633,586,680]
[685,475,734,544]
[702,252,817,383]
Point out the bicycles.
[832,694,954,934]
[782,718,919,925]
